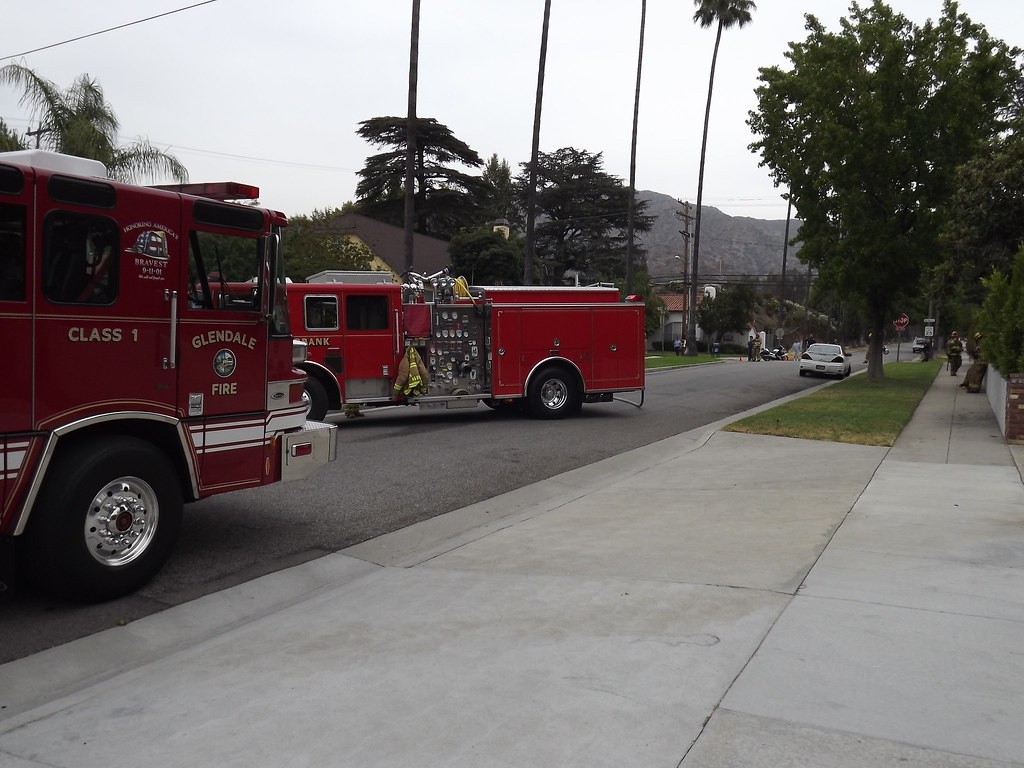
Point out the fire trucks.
[191,266,645,422]
[1,148,341,599]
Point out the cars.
[799,343,852,379]
[912,337,926,353]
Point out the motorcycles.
[761,345,788,361]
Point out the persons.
[747,334,762,362]
[920,339,931,362]
[863,332,889,363]
[805,333,817,349]
[832,338,840,345]
[792,339,801,362]
[958,332,988,393]
[674,337,682,356]
[946,331,963,376]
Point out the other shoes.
[951,372,956,376]
[862,361,868,364]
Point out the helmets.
[974,332,981,340]
[950,331,958,337]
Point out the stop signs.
[894,313,909,328]
